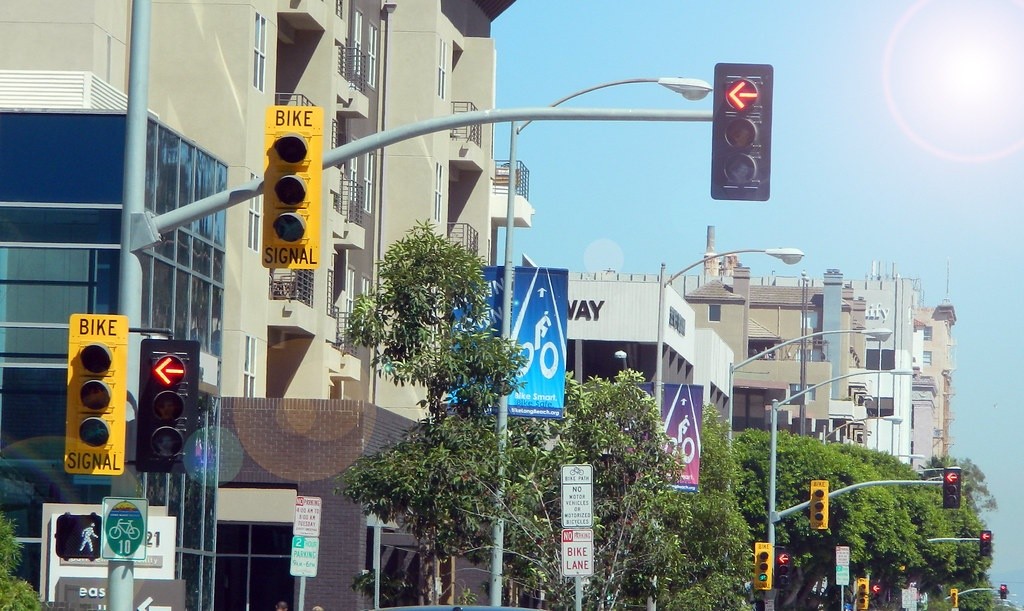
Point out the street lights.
[764,369,913,611]
[647,247,804,611]
[822,416,902,445]
[490,78,713,606]
[724,328,893,610]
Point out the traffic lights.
[753,542,773,591]
[870,580,881,600]
[712,63,773,201]
[810,479,829,529]
[136,338,202,473]
[1000,584,1007,599]
[773,546,792,590]
[262,107,324,270]
[979,530,991,556]
[951,589,958,607]
[54,512,102,562]
[63,313,130,476]
[943,468,962,508]
[856,578,869,610]
